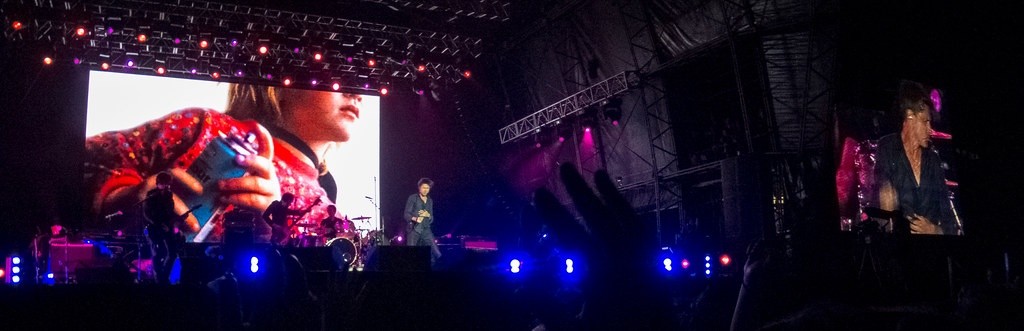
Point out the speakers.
[363,245,432,272]
[51,242,94,285]
[79,254,124,284]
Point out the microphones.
[105,211,124,219]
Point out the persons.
[834,87,962,235]
[322,205,344,242]
[79,162,761,331]
[143,173,189,288]
[85,81,361,242]
[263,193,312,244]
[404,178,441,260]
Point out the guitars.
[165,203,203,238]
[271,197,322,244]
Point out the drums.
[326,236,357,265]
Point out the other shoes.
[163,268,170,282]
[154,271,163,284]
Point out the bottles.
[183,129,259,241]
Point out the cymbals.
[352,217,372,220]
[296,223,318,227]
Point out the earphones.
[908,115,913,120]
